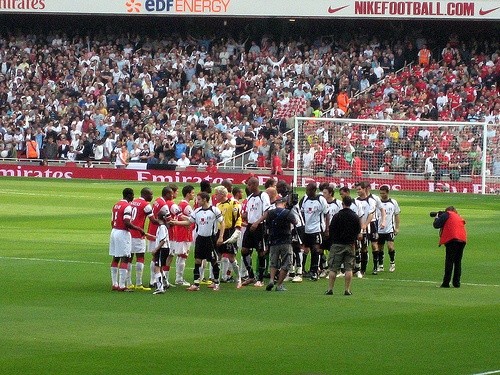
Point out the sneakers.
[127,284,135,290]
[175,280,190,286]
[345,290,352,295]
[200,278,212,284]
[134,286,151,291]
[113,285,118,290]
[284,271,366,282]
[168,282,176,288]
[325,290,333,295]
[153,288,164,294]
[205,277,265,291]
[276,286,287,291]
[149,283,155,288]
[389,265,395,272]
[187,285,199,291]
[118,286,128,291]
[163,280,169,290]
[377,267,384,271]
[372,271,377,274]
[266,282,274,291]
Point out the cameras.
[430,211,444,217]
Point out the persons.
[324,196,361,295]
[0,12,279,172]
[271,151,282,174]
[108,182,190,295]
[175,175,305,292]
[433,206,466,287]
[279,20,500,181]
[299,181,351,282]
[352,182,401,276]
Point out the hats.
[275,197,286,204]
